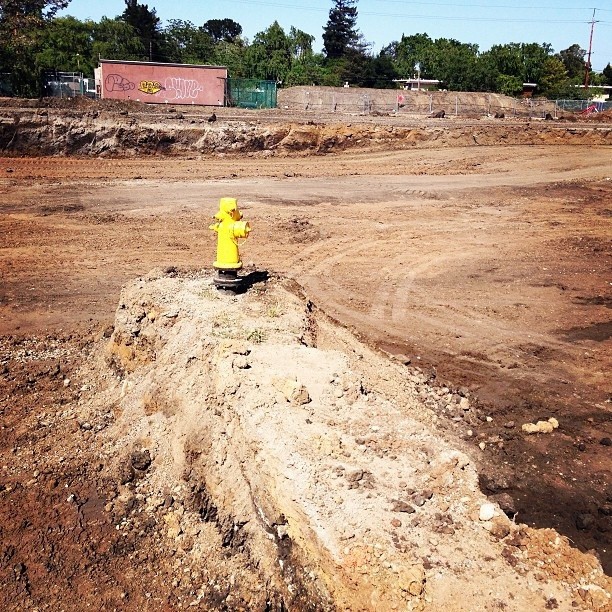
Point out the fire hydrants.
[209,198,253,290]
